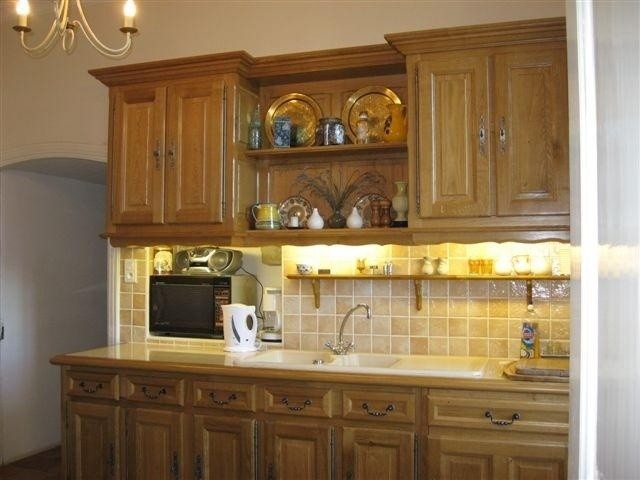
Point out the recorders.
[174,247,243,275]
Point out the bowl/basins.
[297,261,316,277]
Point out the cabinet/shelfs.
[381,14,570,245]
[243,42,408,244]
[59,365,121,478]
[88,49,259,247]
[257,379,336,479]
[424,387,569,479]
[120,370,258,480]
[337,385,424,479]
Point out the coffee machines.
[254,285,288,344]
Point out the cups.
[350,246,572,275]
[249,201,280,230]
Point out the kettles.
[219,303,262,352]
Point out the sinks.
[332,355,401,369]
[233,349,332,368]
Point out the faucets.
[327,304,371,355]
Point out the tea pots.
[380,102,409,146]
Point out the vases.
[346,207,365,228]
[391,180,409,221]
[329,211,345,227]
[307,208,325,229]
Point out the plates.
[342,82,408,143]
[278,195,312,229]
[263,93,322,148]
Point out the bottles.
[246,102,264,150]
[356,111,371,143]
[517,303,545,359]
[370,200,380,225]
[379,196,393,226]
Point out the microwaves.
[146,272,257,343]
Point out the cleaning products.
[521,305,541,359]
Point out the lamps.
[10,0,141,60]
